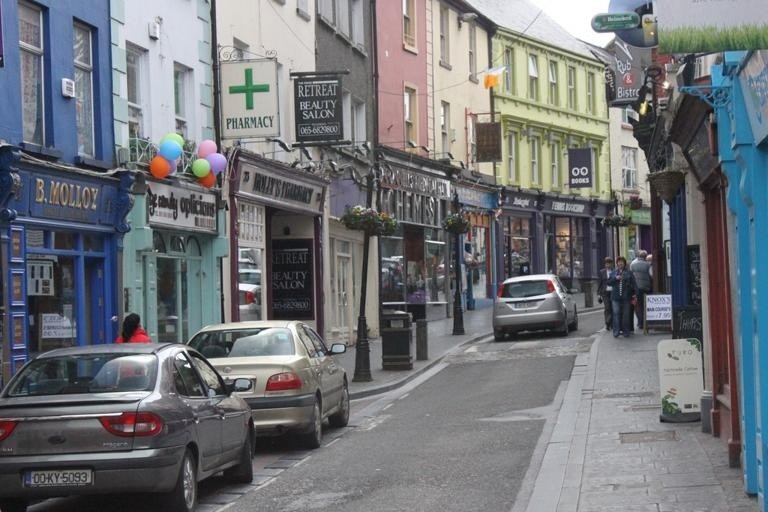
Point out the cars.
[187,318,350,449]
[235,267,262,323]
[0,342,256,512]
[157,312,185,343]
[492,273,578,342]
[381,250,528,304]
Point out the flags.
[483,67,506,90]
[567,148,594,188]
[292,74,345,141]
[475,122,502,163]
[463,230,473,259]
[612,36,643,103]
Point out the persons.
[606,256,639,339]
[112,312,153,389]
[645,254,652,263]
[629,249,654,331]
[595,256,615,331]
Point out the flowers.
[341,204,400,236]
[442,214,470,234]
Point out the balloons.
[149,134,227,188]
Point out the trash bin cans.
[379,309,414,370]
